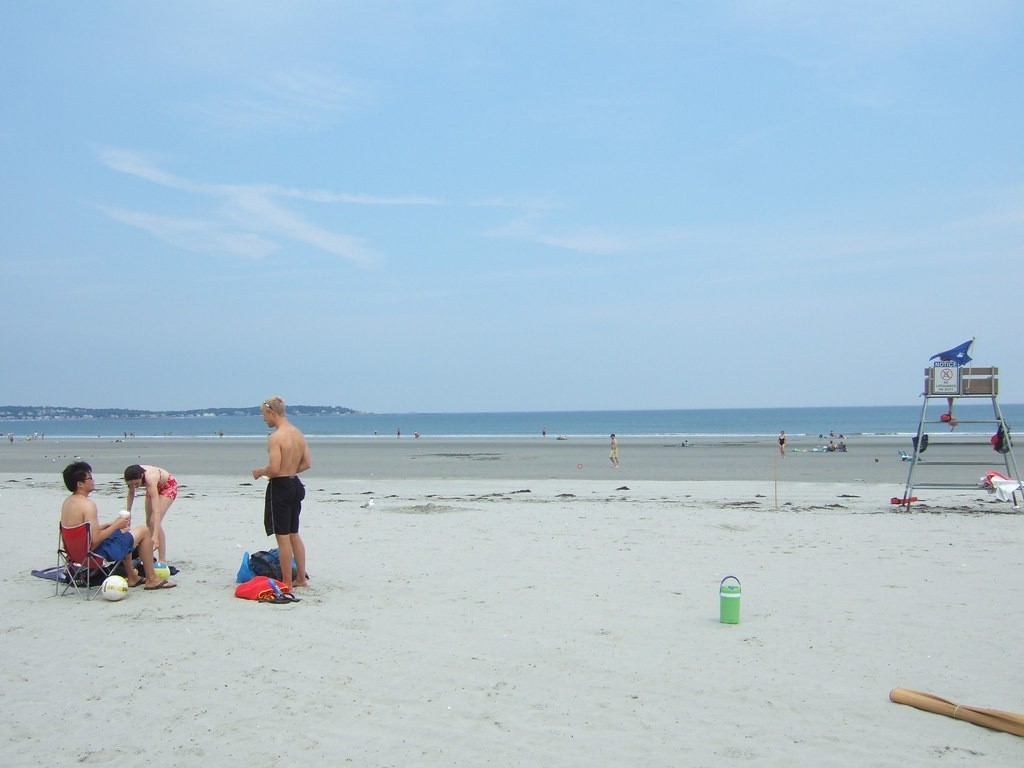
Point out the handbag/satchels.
[234,576,289,600]
[912,422,929,453]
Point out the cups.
[119,510,130,531]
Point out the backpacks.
[995,417,1014,454]
[250,549,299,582]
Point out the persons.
[838,441,846,452]
[253,396,311,593]
[397,426,400,438]
[219,428,223,437]
[268,429,271,436]
[557,435,567,440]
[610,434,619,468]
[0,431,38,444]
[828,440,836,451]
[124,465,178,563]
[414,430,419,439]
[819,434,823,438]
[777,430,787,458]
[838,433,845,439]
[829,430,835,438]
[61,462,178,590]
[543,427,546,438]
[124,431,135,439]
[680,440,688,446]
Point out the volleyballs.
[102,575,130,602]
[152,561,172,583]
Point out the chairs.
[55,521,121,601]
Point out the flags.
[929,339,972,366]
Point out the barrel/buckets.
[719,575,741,623]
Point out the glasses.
[263,400,274,411]
[84,476,92,481]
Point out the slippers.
[278,593,301,602]
[144,580,177,590]
[129,576,146,587]
[259,594,289,604]
